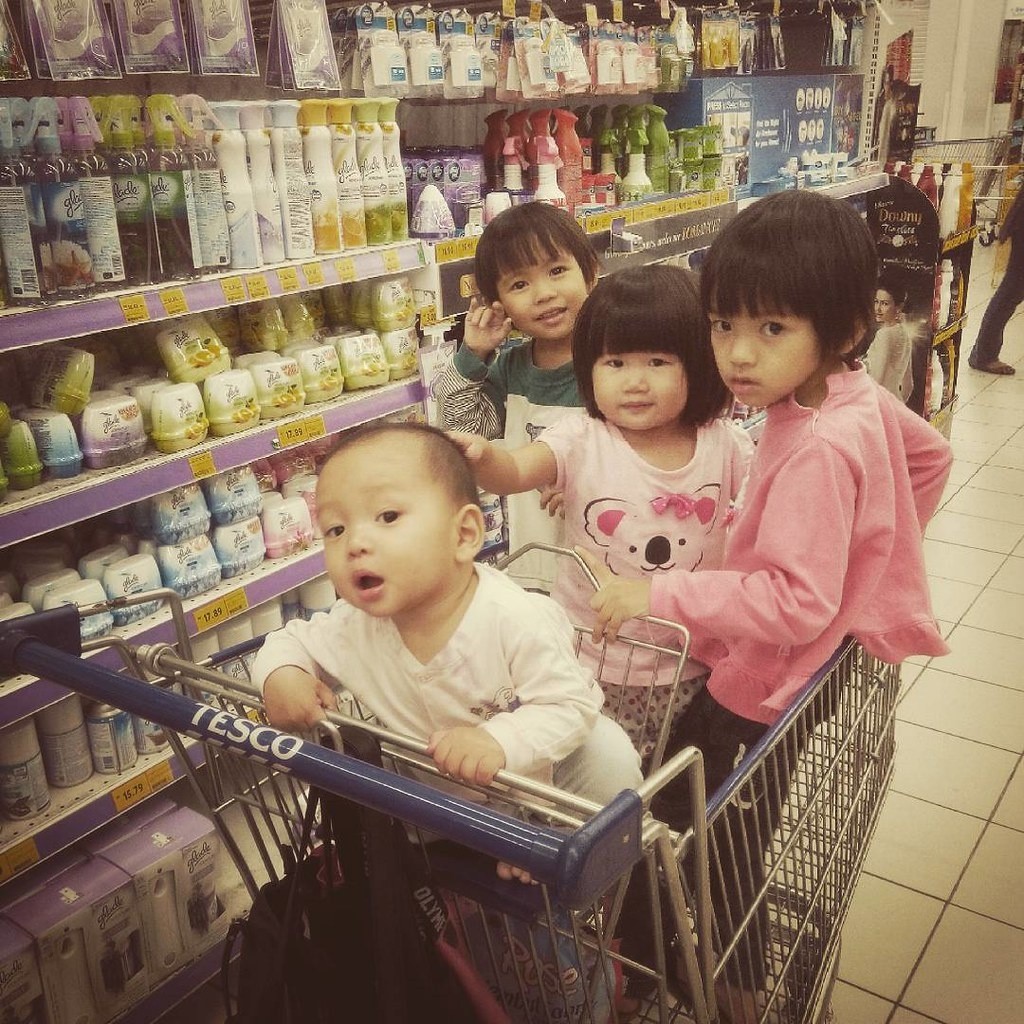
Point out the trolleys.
[912,129,1014,246]
[0,539,902,1024]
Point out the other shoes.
[970,360,1015,375]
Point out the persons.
[878,64,898,174]
[444,263,758,1013]
[572,190,953,1024]
[866,276,914,407]
[251,421,645,885]
[967,184,1024,376]
[433,203,605,599]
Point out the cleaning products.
[930,257,967,421]
[887,158,976,239]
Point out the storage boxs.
[0,915,48,1024]
[655,67,833,201]
[101,805,231,981]
[4,856,150,1024]
[0,843,90,913]
[85,793,177,855]
[834,71,867,159]
[750,178,797,199]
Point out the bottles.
[82,573,341,776]
[930,339,956,415]
[931,259,967,329]
[1,718,52,822]
[884,160,977,242]
[37,692,94,787]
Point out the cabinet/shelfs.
[0,0,973,1024]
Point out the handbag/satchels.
[222,727,448,1024]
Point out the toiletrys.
[404,101,725,242]
[2,91,411,313]
